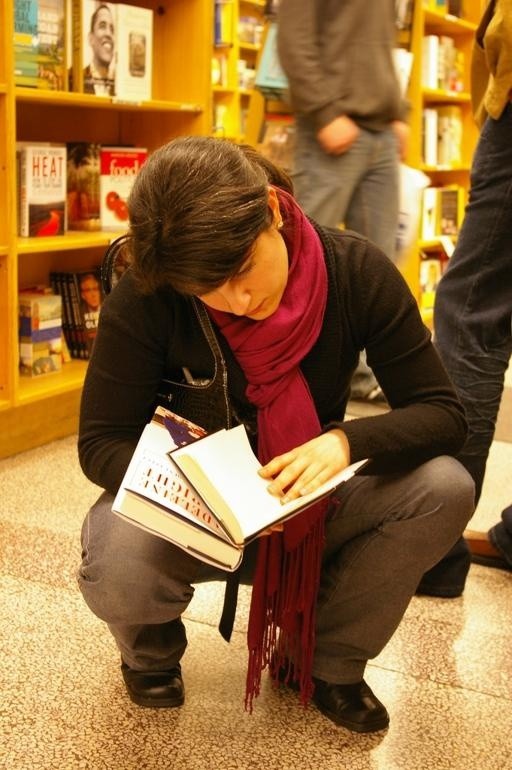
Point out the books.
[17,140,147,238]
[168,424,377,552]
[18,269,102,378]
[112,402,248,575]
[12,0,151,100]
[393,2,467,310]
[211,0,297,169]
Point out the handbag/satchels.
[98,233,249,436]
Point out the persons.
[412,0,511,600]
[276,1,408,403]
[70,133,483,739]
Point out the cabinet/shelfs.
[0,1,214,462]
[215,1,420,302]
[420,0,485,310]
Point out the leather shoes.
[463,528,505,567]
[267,650,390,735]
[119,663,187,708]
[415,550,471,598]
